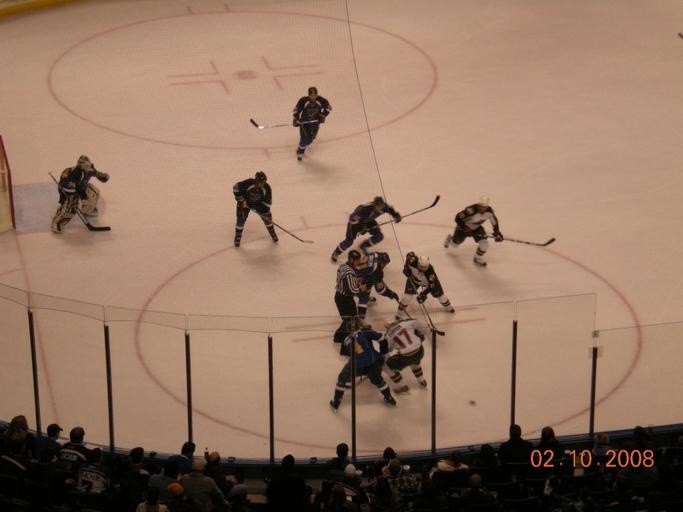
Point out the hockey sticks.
[48,171,111,231]
[247,207,314,243]
[250,118,318,129]
[408,276,445,336]
[476,234,555,246]
[359,196,440,234]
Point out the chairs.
[0,451,682,510]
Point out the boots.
[329,388,345,410]
[411,368,427,387]
[474,245,487,266]
[358,305,372,328]
[440,298,455,313]
[331,244,344,263]
[395,302,408,320]
[359,239,373,255]
[389,372,409,393]
[380,386,397,405]
[445,234,458,249]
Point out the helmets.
[346,320,359,333]
[77,155,91,172]
[384,319,394,329]
[373,196,386,205]
[348,251,360,258]
[378,253,390,263]
[308,87,318,95]
[419,256,430,267]
[478,196,490,207]
[255,171,267,181]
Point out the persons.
[0,406,682,512]
[291,86,333,162]
[49,155,110,235]
[380,314,428,394]
[330,195,402,262]
[330,250,362,339]
[394,249,455,318]
[356,251,397,321]
[230,170,278,248]
[328,316,396,410]
[443,195,504,267]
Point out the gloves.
[319,109,329,123]
[393,212,401,223]
[293,110,300,126]
[493,232,503,242]
[257,202,270,214]
[235,196,248,209]
[463,226,472,236]
[387,291,399,299]
[417,292,427,303]
[357,225,367,234]
[403,265,411,276]
